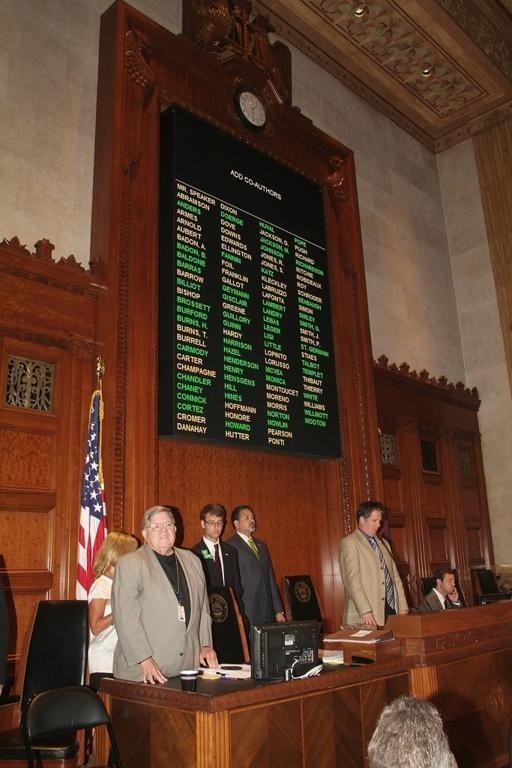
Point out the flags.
[65,392,106,600]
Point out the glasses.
[203,519,227,527]
[145,522,176,528]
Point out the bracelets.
[107,614,114,626]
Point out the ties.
[368,536,396,610]
[214,544,223,583]
[247,537,261,562]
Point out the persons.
[418,567,464,612]
[337,500,410,627]
[111,504,221,685]
[188,502,251,662]
[85,532,140,692]
[366,694,460,768]
[223,504,288,623]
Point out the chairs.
[280,574,327,635]
[19,681,122,768]
[469,567,499,601]
[419,569,469,607]
[1,596,92,766]
[206,585,251,665]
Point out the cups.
[180,671,198,692]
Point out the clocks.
[235,86,269,135]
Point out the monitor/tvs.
[250,619,319,680]
